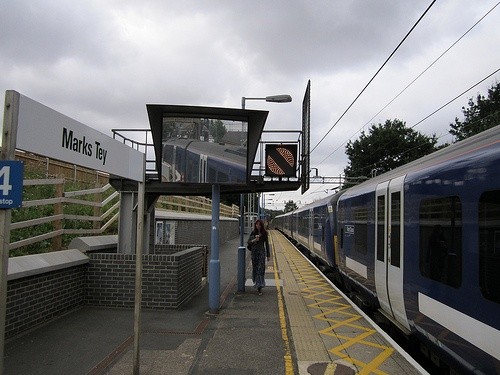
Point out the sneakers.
[258,287,262,296]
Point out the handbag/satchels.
[247,244,252,251]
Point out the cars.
[176,134,226,145]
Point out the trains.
[270,121,500,375]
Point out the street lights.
[260,192,274,226]
[237,94,294,294]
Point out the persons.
[247,219,271,296]
